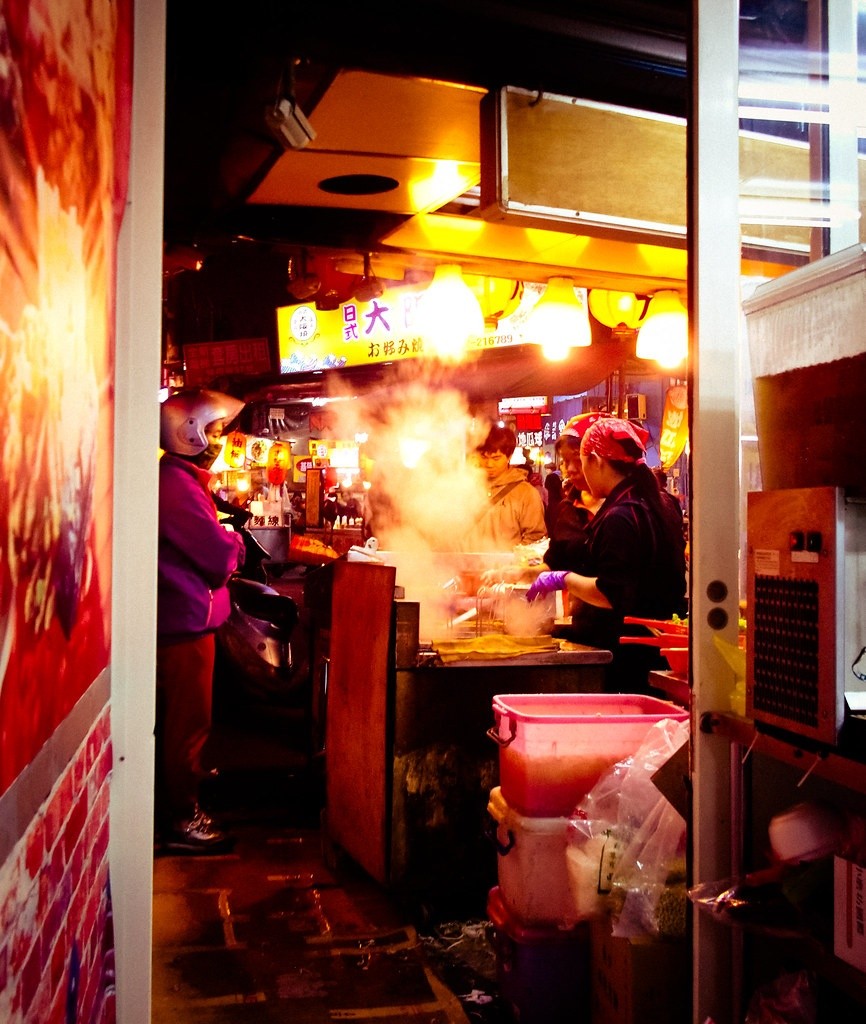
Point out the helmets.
[159,388,246,456]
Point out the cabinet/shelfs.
[719,242,866,1024]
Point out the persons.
[477,410,688,698]
[160,389,245,843]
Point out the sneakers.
[154,812,240,856]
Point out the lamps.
[423,264,690,365]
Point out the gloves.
[525,571,572,604]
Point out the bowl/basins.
[766,799,843,866]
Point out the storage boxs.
[485,886,592,1024]
[493,692,691,816]
[488,786,589,928]
[833,855,866,973]
[590,916,693,1024]
[754,352,866,500]
